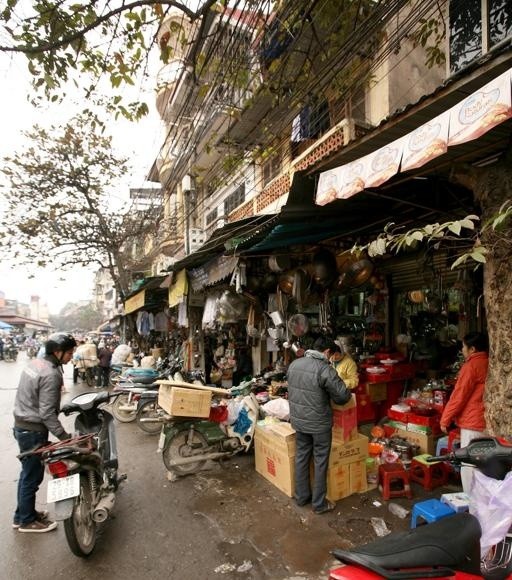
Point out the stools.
[380,436,471,529]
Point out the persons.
[329,344,359,390]
[10,332,77,533]
[287,335,352,513]
[439,330,489,493]
[0,329,112,389]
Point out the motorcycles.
[34,388,132,560]
[1,333,44,363]
[72,339,276,479]
[326,434,511,580]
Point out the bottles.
[367,458,378,484]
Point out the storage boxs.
[255,406,370,502]
[158,385,212,419]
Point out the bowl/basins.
[368,442,401,463]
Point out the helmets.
[46,334,76,355]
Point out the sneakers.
[315,501,335,514]
[12,510,49,528]
[18,517,57,533]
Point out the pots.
[394,437,420,461]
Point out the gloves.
[57,431,71,443]
